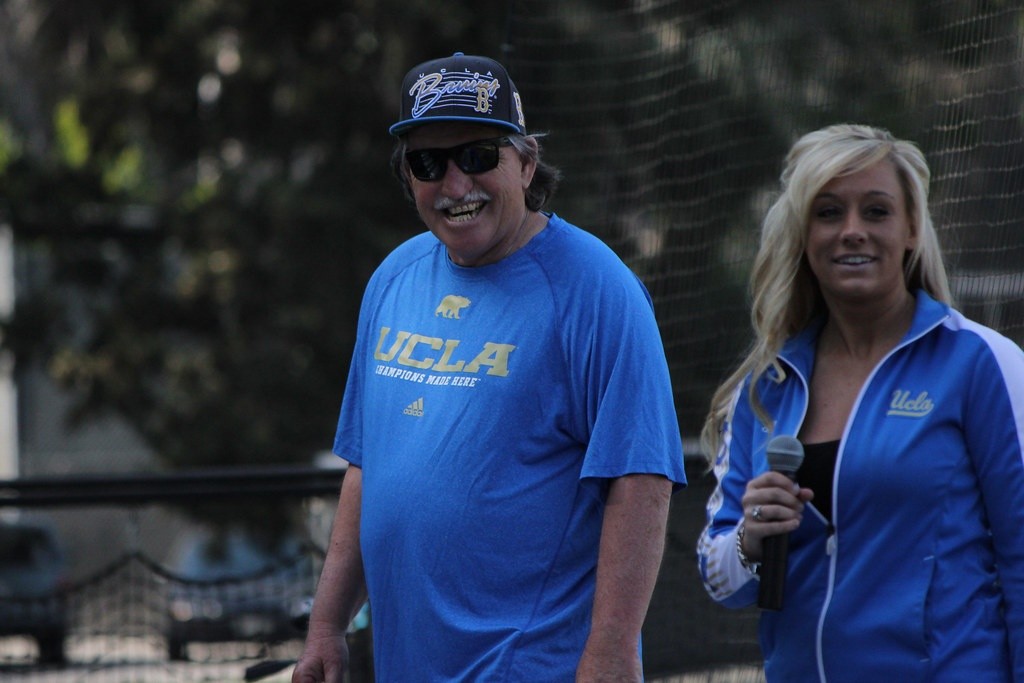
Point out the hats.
[388,52,533,140]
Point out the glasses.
[405,139,505,181]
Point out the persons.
[290,52,689,683]
[696,124,1024,683]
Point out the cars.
[1,521,75,672]
[160,528,319,661]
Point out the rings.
[753,505,763,522]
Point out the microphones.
[757,436,805,611]
